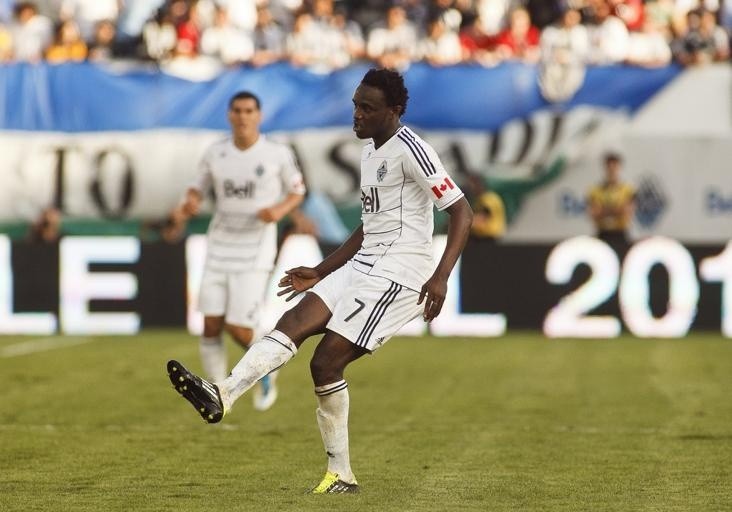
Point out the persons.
[181,90,307,412]
[167,66,474,495]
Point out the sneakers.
[253,369,279,411]
[306,471,359,495]
[167,359,224,422]
